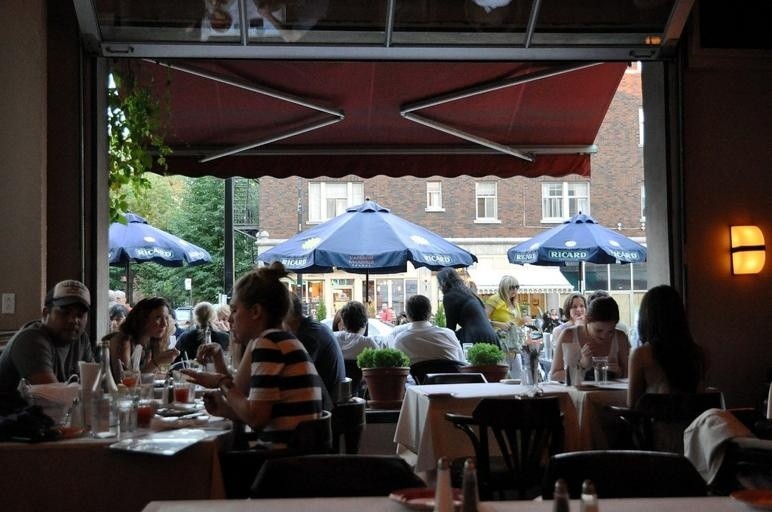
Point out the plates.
[61,422,92,440]
[389,487,464,511]
[156,406,199,417]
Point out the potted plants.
[358,347,410,408]
[465,342,509,382]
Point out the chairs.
[331,395,366,437]
[220,411,334,498]
[598,389,721,450]
[445,396,565,502]
[424,372,488,383]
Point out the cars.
[319,318,394,336]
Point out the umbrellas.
[108,213,213,306]
[254,195,478,304]
[507,210,647,293]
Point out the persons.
[548,290,629,379]
[281,289,472,399]
[627,283,707,453]
[553,292,587,340]
[436,267,503,352]
[484,275,531,385]
[1,279,229,442]
[180,261,322,450]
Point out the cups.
[592,356,609,385]
[462,342,473,361]
[118,370,153,432]
[172,382,190,403]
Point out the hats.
[45,280,92,312]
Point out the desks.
[392,381,579,484]
[0,359,236,512]
[570,378,631,449]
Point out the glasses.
[508,285,520,290]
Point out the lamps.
[730,225,765,275]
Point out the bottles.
[70,341,118,438]
[162,379,173,403]
[435,458,598,511]
[202,330,216,372]
